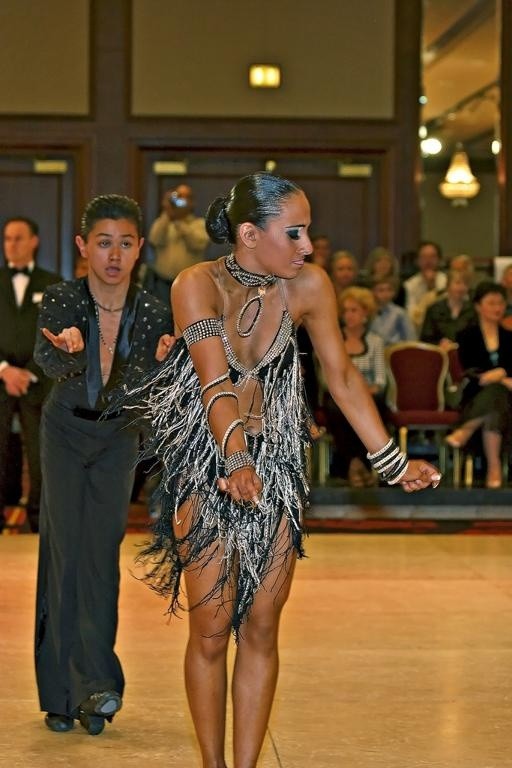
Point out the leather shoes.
[484,479,502,489]
[44,711,75,732]
[444,434,464,448]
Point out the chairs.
[299,337,512,489]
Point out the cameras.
[170,192,187,208]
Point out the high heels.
[77,692,123,734]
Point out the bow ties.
[8,266,33,279]
